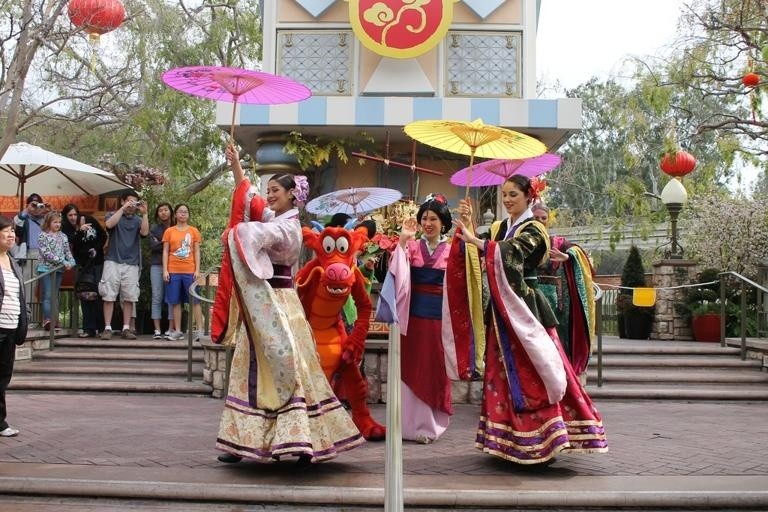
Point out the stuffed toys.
[294,226,385,441]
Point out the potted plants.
[674,268,731,342]
[615,246,655,339]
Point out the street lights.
[649,177,699,338]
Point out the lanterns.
[659,149,696,182]
[742,72,759,88]
[68,0,124,74]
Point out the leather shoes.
[78,332,89,338]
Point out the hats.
[26,193,42,204]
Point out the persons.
[531,205,595,376]
[374,194,452,444]
[211,141,366,471]
[450,175,609,469]
[0,214,27,437]
[14,191,204,340]
[323,213,380,409]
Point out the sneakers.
[43,320,62,331]
[152,330,201,342]
[217,452,242,464]
[101,329,113,340]
[0,427,19,437]
[120,329,138,340]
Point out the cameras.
[35,203,45,210]
[132,200,141,208]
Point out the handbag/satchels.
[74,281,99,301]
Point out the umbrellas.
[161,66,313,144]
[0,142,135,213]
[450,154,561,188]
[304,186,402,220]
[403,118,548,201]
